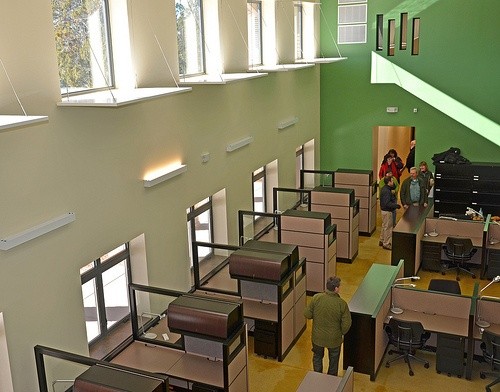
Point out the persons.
[416,160,433,195]
[302,275,352,376]
[378,169,400,227]
[378,177,402,250]
[379,153,402,185]
[399,167,428,208]
[406,140,416,175]
[381,147,405,182]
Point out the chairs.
[479,329,500,392]
[441,237,478,283]
[428,278,462,295]
[385,318,432,375]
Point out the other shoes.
[379,241,391,246]
[383,243,392,250]
